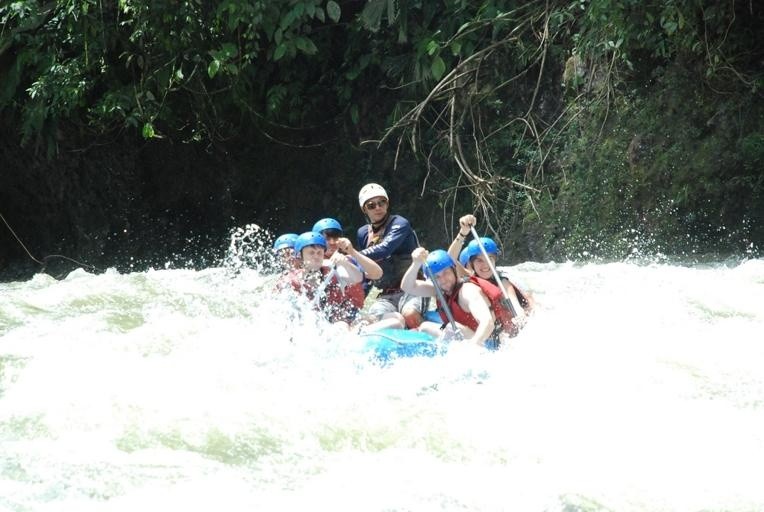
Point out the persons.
[268,183,535,350]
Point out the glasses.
[365,198,387,210]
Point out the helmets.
[459,237,498,268]
[358,183,389,214]
[422,249,456,280]
[273,218,343,260]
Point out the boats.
[350,308,502,372]
